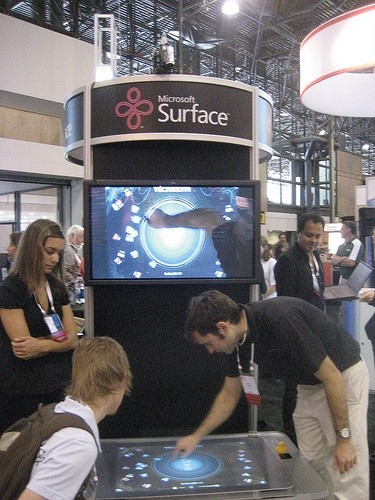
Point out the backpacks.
[0,402,99,500]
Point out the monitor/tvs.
[80,178,262,286]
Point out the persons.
[4,224,84,305]
[0,336,132,500]
[259,221,362,338]
[172,290,370,500]
[272,213,330,447]
[1,218,79,426]
[357,288,375,359]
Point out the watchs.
[335,428,352,440]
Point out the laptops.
[322,259,374,300]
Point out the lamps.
[300,0,375,117]
